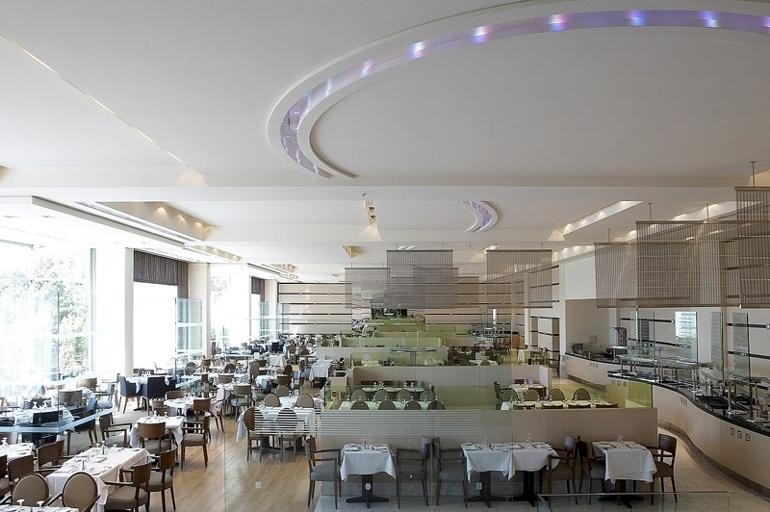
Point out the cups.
[153,410,168,423]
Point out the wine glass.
[372,381,385,390]
[403,382,415,391]
[36,500,45,511]
[346,394,439,404]
[32,401,48,411]
[16,498,26,512]
[0,437,8,452]
[71,440,117,474]
[184,392,190,402]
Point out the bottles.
[549,393,552,402]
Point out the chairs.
[1,328,318,473]
[9,455,56,489]
[304,436,342,509]
[316,328,621,410]
[539,435,578,507]
[103,461,154,512]
[395,435,432,509]
[0,453,15,504]
[433,436,470,509]
[577,434,618,506]
[131,444,179,511]
[634,433,678,505]
[0,473,51,506]
